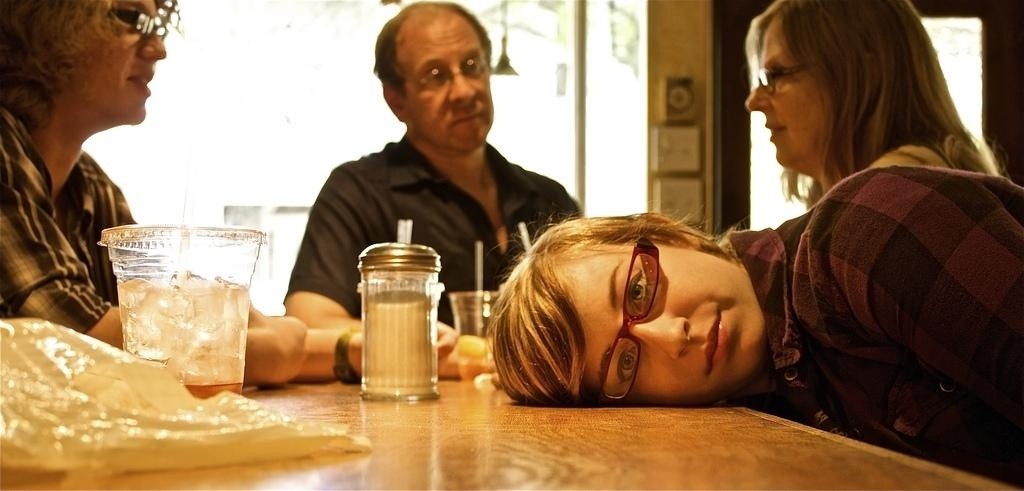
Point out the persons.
[0,0,457,386]
[492,165,1019,479]
[719,1,1001,211]
[284,1,583,379]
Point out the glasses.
[395,53,484,88]
[597,230,659,402]
[85,5,155,36]
[757,58,824,94]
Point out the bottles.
[356,240,444,404]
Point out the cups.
[98,225,266,399]
[449,291,500,381]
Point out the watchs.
[332,327,360,387]
